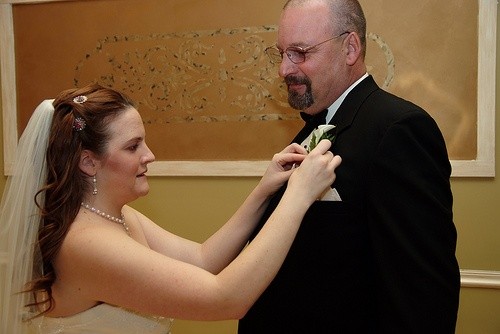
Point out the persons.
[0,87,342,333]
[239,0,461,334]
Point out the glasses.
[265,31,351,64]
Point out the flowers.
[302,124,336,153]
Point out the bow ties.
[300,109,328,135]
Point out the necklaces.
[80,202,128,233]
